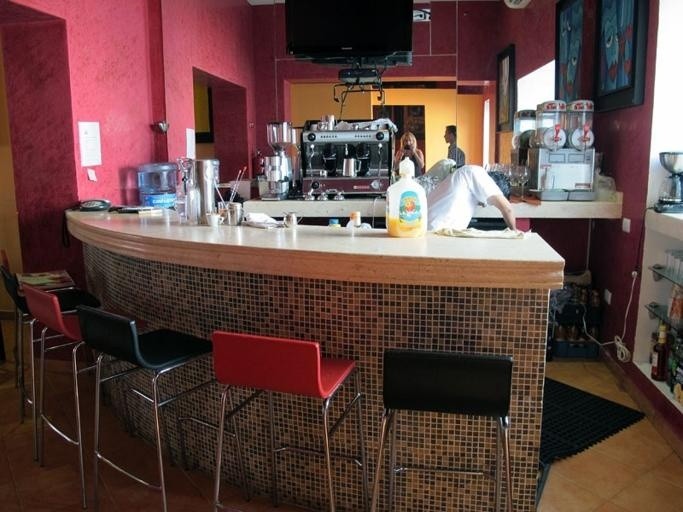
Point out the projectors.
[338,68,381,84]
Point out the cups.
[663,248,682,285]
[205,200,244,226]
[173,195,185,218]
[281,210,303,225]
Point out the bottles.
[385,156,429,237]
[175,156,202,225]
[648,283,682,407]
[346,211,361,228]
[328,218,341,227]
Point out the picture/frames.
[555,0,582,128]
[589,0,652,115]
[494,43,516,135]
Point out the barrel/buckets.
[136,163,176,208]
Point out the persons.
[394,132,425,178]
[426,165,517,232]
[443,125,465,168]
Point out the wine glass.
[485,162,530,201]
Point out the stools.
[1,266,173,512]
[72,305,250,511]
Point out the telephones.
[78,198,111,211]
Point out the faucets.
[579,125,590,151]
[554,125,561,149]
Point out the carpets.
[538,375,647,464]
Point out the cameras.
[404,145,411,150]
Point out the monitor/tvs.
[285,0,414,65]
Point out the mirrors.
[254,0,561,182]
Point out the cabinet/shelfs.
[631,209,682,442]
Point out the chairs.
[210,329,370,512]
[369,347,515,511]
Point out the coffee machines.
[299,118,396,199]
[652,151,683,214]
[257,120,299,200]
